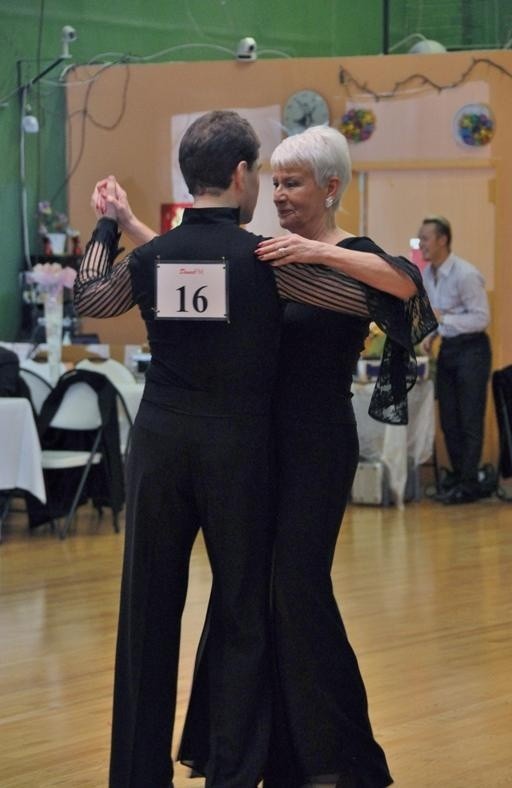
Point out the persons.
[73,108,387,788]
[89,124,438,788]
[418,216,492,504]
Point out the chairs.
[0,344,141,539]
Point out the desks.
[350,379,440,506]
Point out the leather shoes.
[432,476,480,503]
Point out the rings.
[280,248,288,257]
